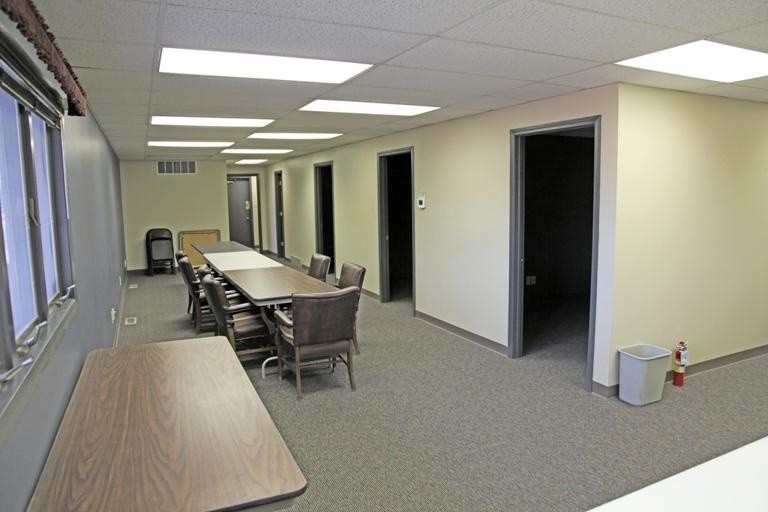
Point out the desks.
[191,240,253,254]
[23,336,309,511]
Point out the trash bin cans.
[618,344,673,406]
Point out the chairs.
[146,229,173,277]
[175,250,366,401]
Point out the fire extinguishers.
[672,341,687,386]
